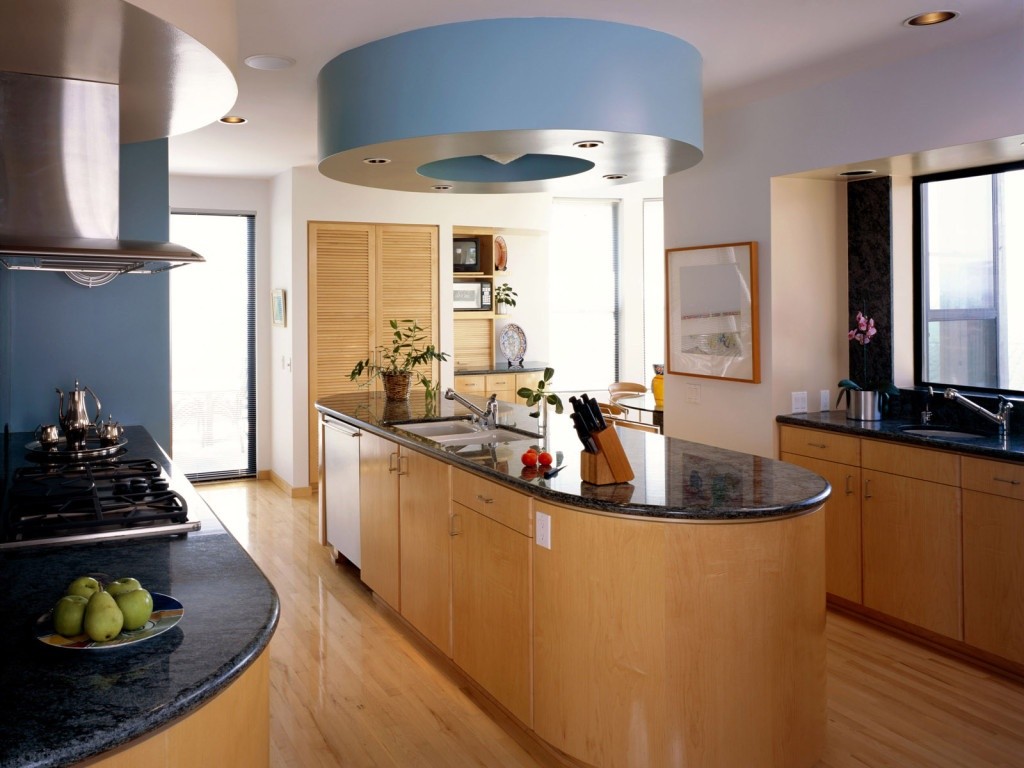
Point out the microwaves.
[453,280,492,311]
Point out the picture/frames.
[663,239,763,384]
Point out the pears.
[84,581,124,642]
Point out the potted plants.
[345,318,449,407]
[494,283,517,313]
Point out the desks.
[618,395,665,432]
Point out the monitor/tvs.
[453,238,480,271]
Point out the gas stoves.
[1,457,201,549]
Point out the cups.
[36,423,60,450]
[100,414,124,445]
[67,427,88,451]
[537,395,548,427]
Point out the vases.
[844,384,886,422]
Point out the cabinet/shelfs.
[777,408,1024,680]
[314,409,543,735]
[456,360,548,405]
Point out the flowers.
[847,309,879,389]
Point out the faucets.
[943,386,1014,438]
[444,386,499,429]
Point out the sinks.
[389,415,545,447]
[896,425,989,441]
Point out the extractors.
[0,0,206,279]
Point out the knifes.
[568,394,607,456]
[544,464,567,480]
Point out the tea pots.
[54,379,102,438]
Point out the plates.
[502,324,527,362]
[495,235,507,272]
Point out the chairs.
[608,380,648,423]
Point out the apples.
[521,449,552,466]
[116,588,153,630]
[69,577,101,601]
[53,595,89,637]
[106,577,143,600]
